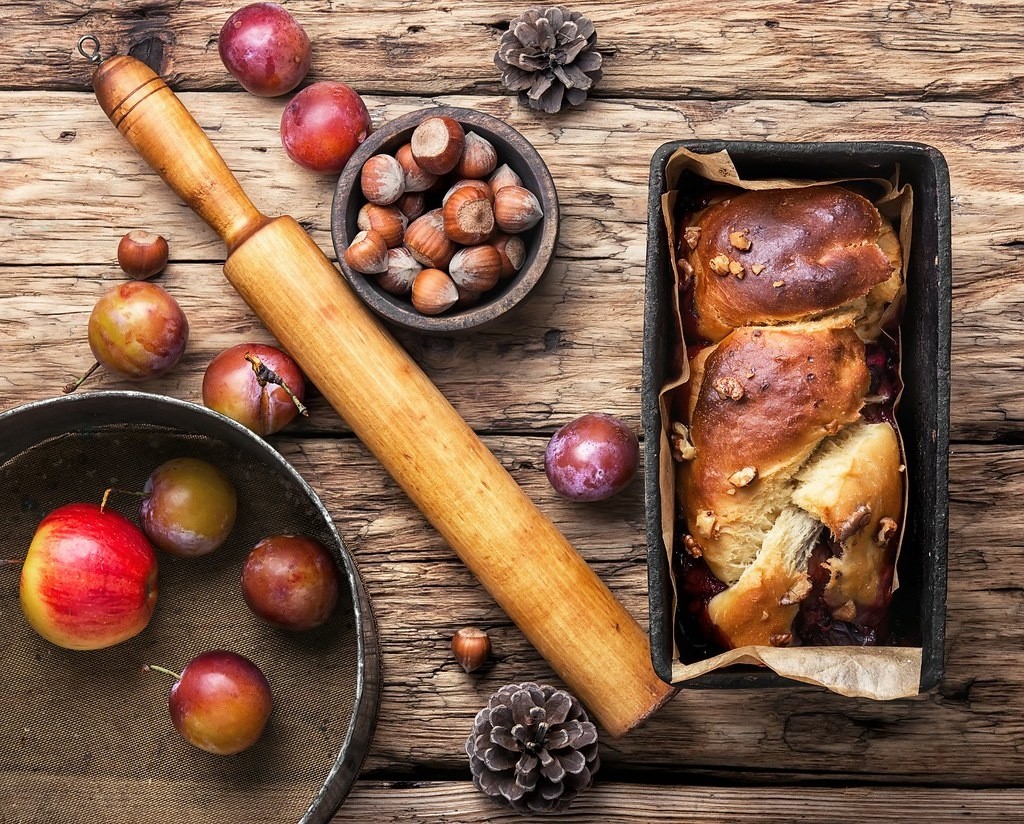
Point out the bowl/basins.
[329,106,562,331]
[638,139,956,692]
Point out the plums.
[143,651,275,755]
[62,281,190,394]
[280,80,372,174]
[217,2,312,97]
[202,343,309,438]
[242,534,338,628]
[139,458,237,557]
[544,412,639,502]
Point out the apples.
[0,501,159,651]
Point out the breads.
[675,184,902,651]
